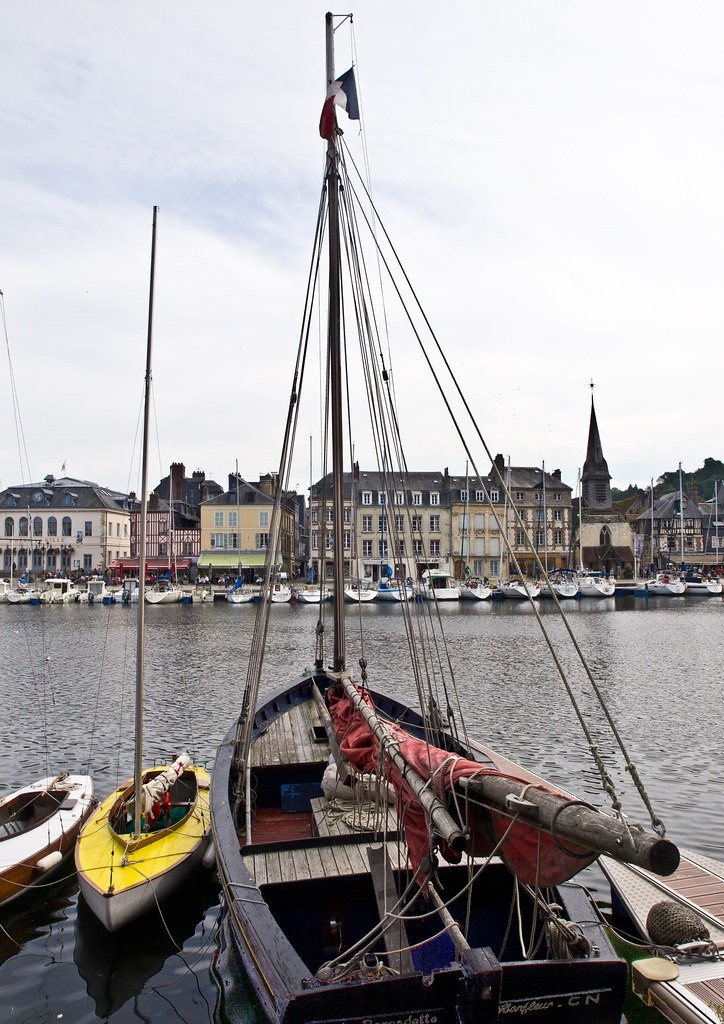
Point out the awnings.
[109,559,190,570]
[198,554,284,568]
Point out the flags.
[319,67,360,140]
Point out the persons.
[18,568,189,592]
[194,572,263,587]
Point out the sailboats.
[210,12,724,1024]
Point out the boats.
[0,205,215,930]
[2,438,616,603]
[633,462,722,595]
